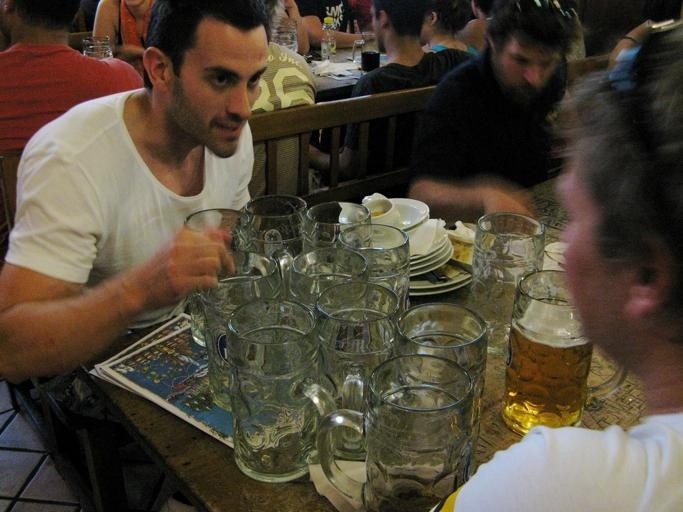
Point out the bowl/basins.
[374,196,431,236]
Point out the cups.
[83,44,113,60]
[181,207,253,349]
[223,295,338,485]
[372,195,402,226]
[351,39,366,66]
[472,211,546,357]
[316,280,402,325]
[286,246,369,315]
[396,299,491,432]
[501,271,630,439]
[267,16,300,54]
[244,192,308,301]
[316,352,476,512]
[313,309,402,463]
[333,223,414,320]
[360,32,381,75]
[300,200,374,253]
[198,255,284,414]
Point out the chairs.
[245,83,441,210]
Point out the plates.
[545,240,572,267]
[404,227,455,277]
[409,263,475,297]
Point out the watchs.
[359,29,370,43]
[613,32,644,46]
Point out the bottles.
[321,16,337,63]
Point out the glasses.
[502,0,580,28]
[596,20,683,257]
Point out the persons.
[244,42,320,195]
[419,0,483,57]
[455,0,492,53]
[423,18,683,511]
[607,20,657,73]
[1,2,147,157]
[0,0,277,385]
[396,3,579,235]
[310,0,478,178]
[89,0,160,80]
